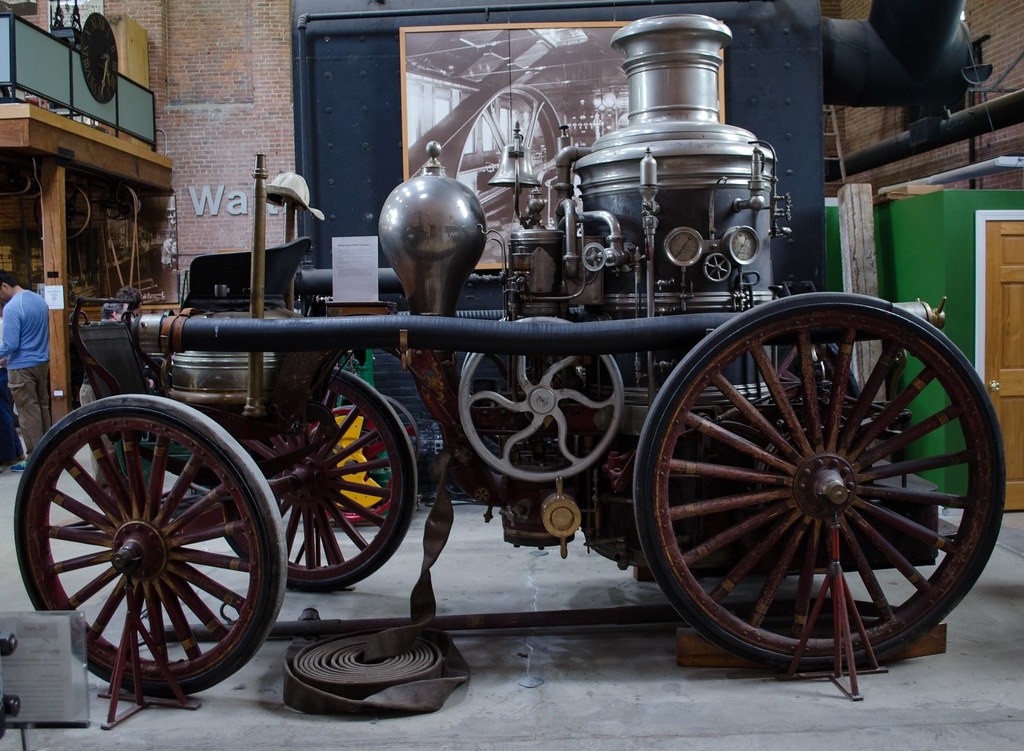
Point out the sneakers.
[10,460,29,472]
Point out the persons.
[0,314,25,468]
[80,286,141,492]
[0,270,50,473]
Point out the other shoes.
[4,454,25,466]
